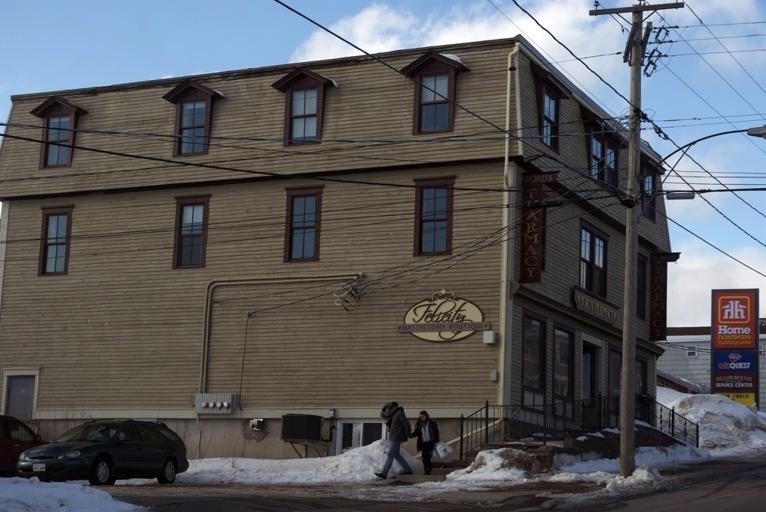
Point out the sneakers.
[424,472,431,475]
[375,473,387,480]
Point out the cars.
[16,416,190,486]
[0,414,50,478]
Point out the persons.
[373,403,411,479]
[407,410,440,476]
